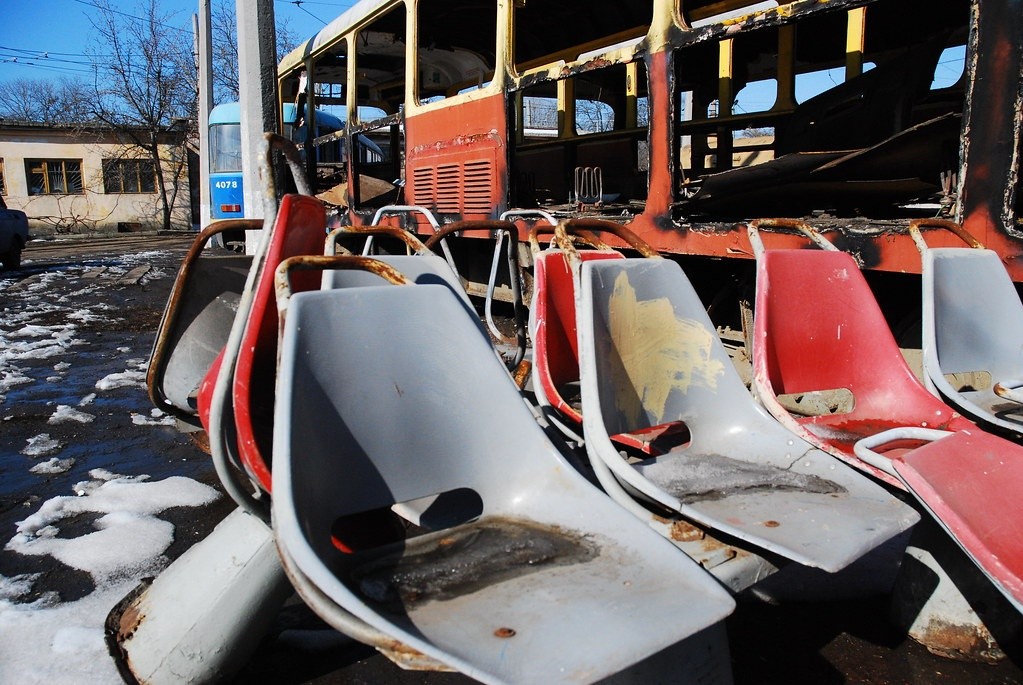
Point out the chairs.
[527,223,626,427]
[908,218,1022,433]
[556,217,924,573]
[853,428,1023,613]
[272,254,737,685]
[748,218,981,492]
[200,131,354,555]
[320,223,492,348]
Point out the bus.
[278,1,1023,349]
[204,100,400,254]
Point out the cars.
[1,193,31,271]
